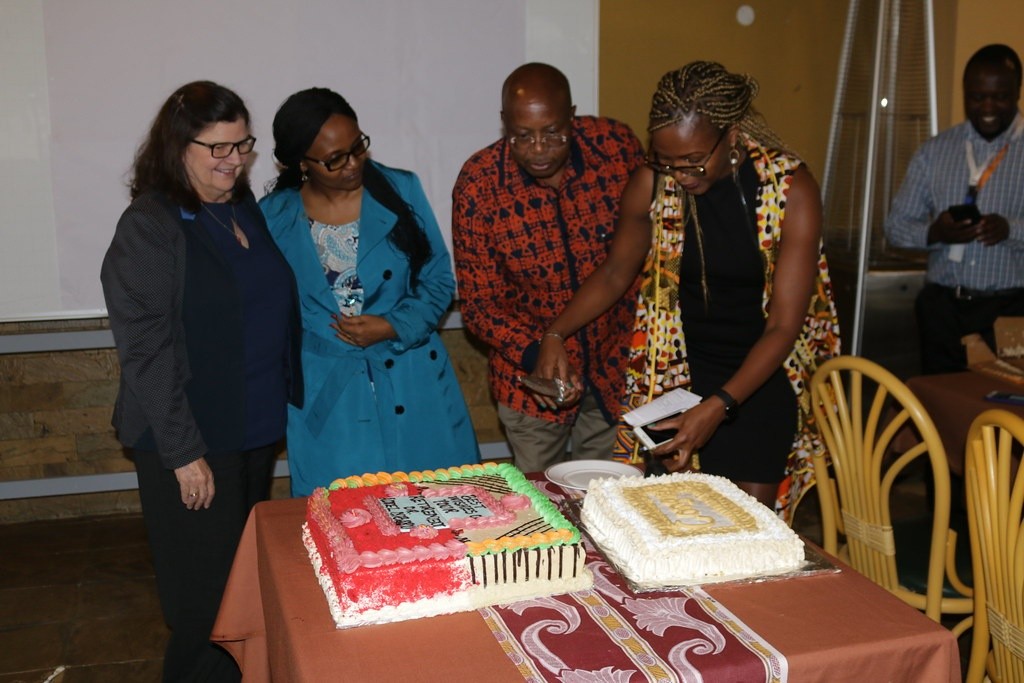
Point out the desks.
[212,463,959,683]
[894,370,1024,481]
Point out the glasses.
[503,113,573,150]
[644,124,731,177]
[190,134,256,158]
[302,132,371,172]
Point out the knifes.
[517,375,564,396]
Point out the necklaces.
[200,202,241,242]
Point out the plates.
[545,460,645,491]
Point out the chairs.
[807,355,974,638]
[964,408,1024,683]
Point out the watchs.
[716,390,737,416]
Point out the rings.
[190,493,197,497]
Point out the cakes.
[580,470,805,584]
[302,462,594,626]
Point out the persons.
[451,62,648,473]
[883,44,1024,596]
[100,80,304,683]
[530,60,841,529]
[256,87,482,497]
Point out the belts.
[927,283,1024,300]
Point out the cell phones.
[984,389,1024,405]
[949,204,980,227]
[633,409,688,450]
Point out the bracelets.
[546,333,563,340]
